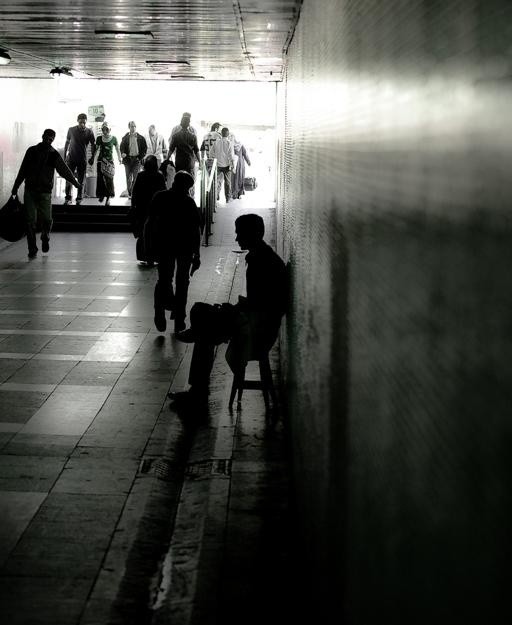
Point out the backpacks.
[100,156,115,179]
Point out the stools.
[227,332,279,418]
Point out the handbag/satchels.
[159,160,175,181]
[1,194,26,242]
[100,151,114,179]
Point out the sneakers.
[99,198,103,202]
[129,195,131,198]
[106,199,109,206]
[226,200,230,202]
[76,199,83,205]
[64,199,72,205]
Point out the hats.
[183,112,191,117]
[42,130,55,140]
[102,122,110,129]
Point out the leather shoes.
[175,323,185,334]
[176,328,195,343]
[168,386,208,403]
[154,309,166,333]
[28,246,38,258]
[42,240,50,252]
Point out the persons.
[158,160,177,190]
[145,170,201,331]
[131,155,166,267]
[168,111,199,161]
[64,114,96,205]
[141,125,168,168]
[94,122,122,206]
[168,214,288,401]
[227,129,251,199]
[11,129,84,257]
[120,121,147,199]
[200,122,223,200]
[209,128,236,203]
[165,117,201,200]
